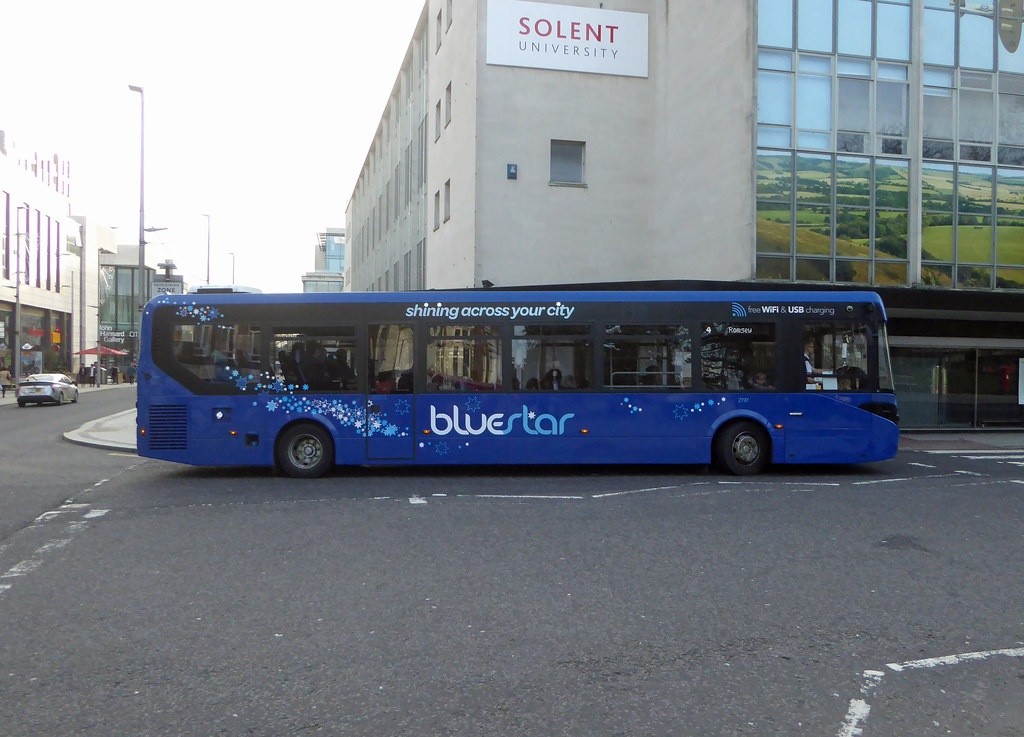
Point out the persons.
[182,335,462,394]
[754,367,769,391]
[0,365,12,399]
[109,365,115,380]
[802,335,835,387]
[79,362,87,388]
[524,377,539,392]
[88,363,96,388]
[609,359,751,395]
[575,376,590,390]
[128,364,137,386]
[510,376,521,391]
[537,368,564,393]
[111,365,120,384]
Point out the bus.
[134,286,902,476]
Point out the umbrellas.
[73,346,129,386]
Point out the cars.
[15,372,80,406]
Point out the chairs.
[235,349,378,395]
